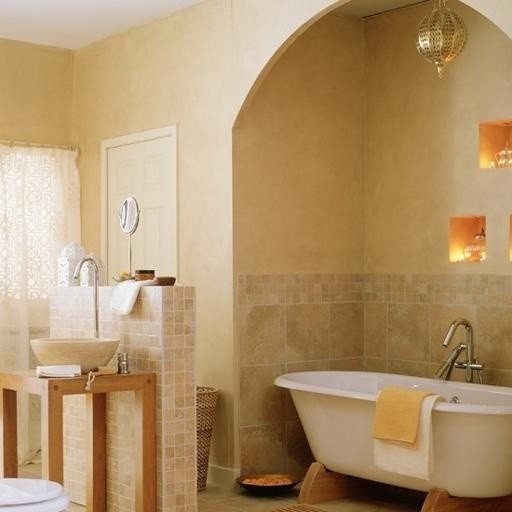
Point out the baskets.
[195,385,220,491]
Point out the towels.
[369,386,449,482]
[37,364,83,380]
[111,279,159,314]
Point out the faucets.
[441,319,473,366]
[74,258,99,337]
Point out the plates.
[234,473,300,492]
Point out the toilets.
[0,478,69,512]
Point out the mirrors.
[118,196,141,235]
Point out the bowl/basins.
[156,276,176,286]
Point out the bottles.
[134,269,156,280]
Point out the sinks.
[30,337,118,372]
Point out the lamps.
[414,2,469,79]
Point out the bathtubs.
[273,371,512,499]
